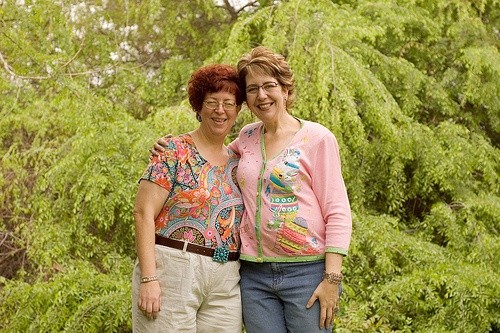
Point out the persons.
[150,45,352,333]
[131,64,246,333]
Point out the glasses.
[245,82,280,94]
[202,99,237,112]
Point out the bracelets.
[324,272,343,286]
[140,275,158,283]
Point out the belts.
[155,235,240,262]
